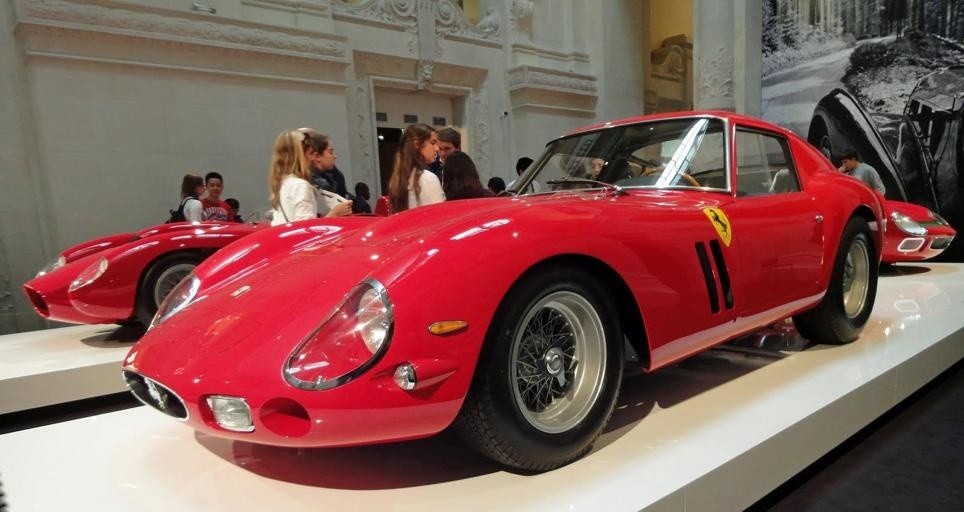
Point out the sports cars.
[23,196,392,328]
[120,109,884,473]
[874,190,955,264]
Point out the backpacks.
[164,198,198,224]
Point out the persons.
[387,122,542,213]
[837,150,888,196]
[892,122,923,201]
[269,126,372,226]
[552,156,605,192]
[180,171,244,222]
